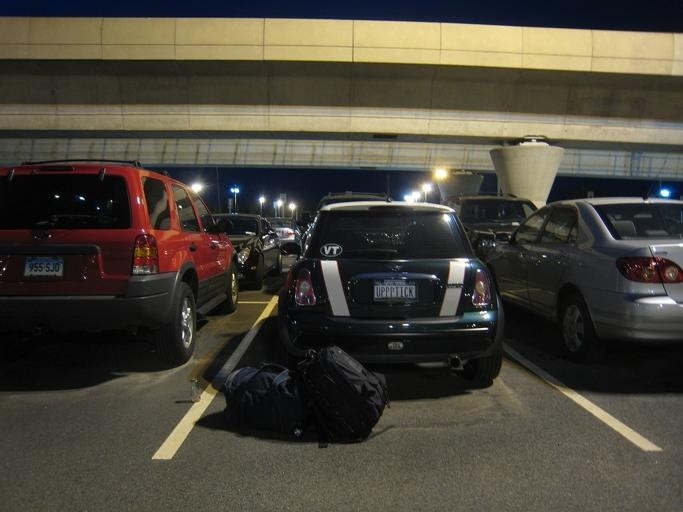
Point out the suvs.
[441,190,540,264]
[297,189,393,258]
[1,158,240,367]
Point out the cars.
[481,195,682,366]
[276,199,502,387]
[210,213,282,290]
[269,217,302,254]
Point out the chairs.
[609,219,637,237]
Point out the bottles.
[190,379,201,402]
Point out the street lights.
[400,181,433,203]
[288,202,296,217]
[276,199,282,217]
[230,184,240,213]
[258,196,266,217]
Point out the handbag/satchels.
[224,360,311,436]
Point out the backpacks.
[288,343,390,448]
